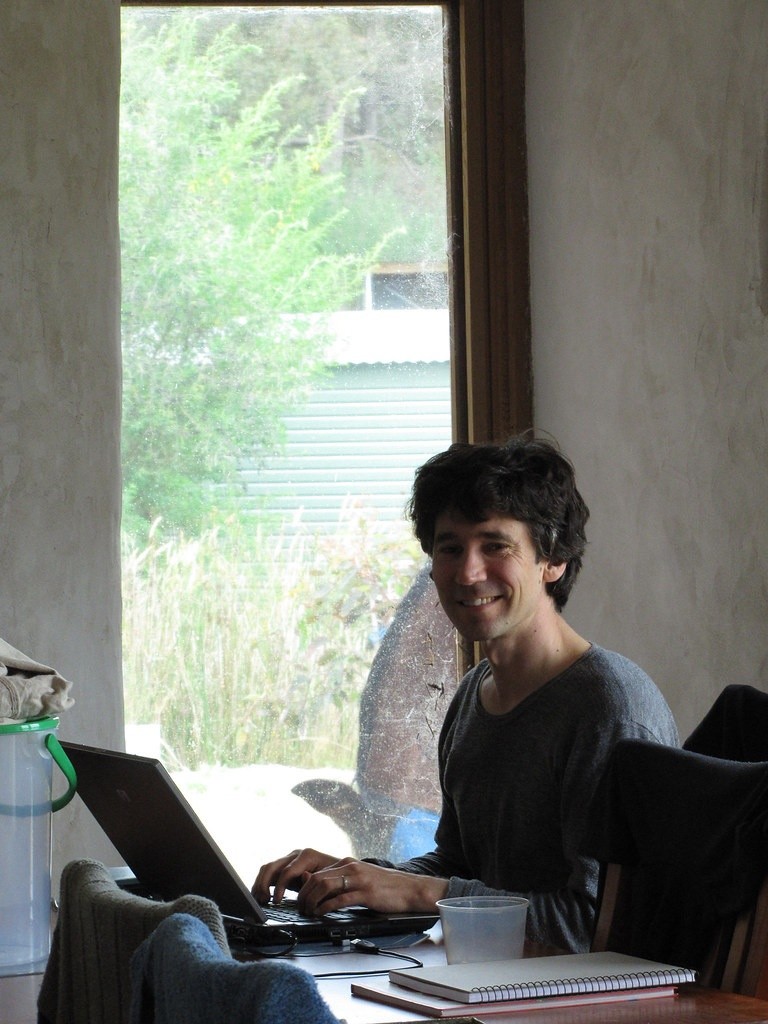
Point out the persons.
[249,429,680,954]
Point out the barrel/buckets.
[0,715,78,977]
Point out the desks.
[228,933,768,1024]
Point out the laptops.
[55,738,440,945]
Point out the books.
[351,953,696,1019]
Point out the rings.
[341,874,348,892]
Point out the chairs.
[37,684,768,1024]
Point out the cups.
[434,895,530,965]
